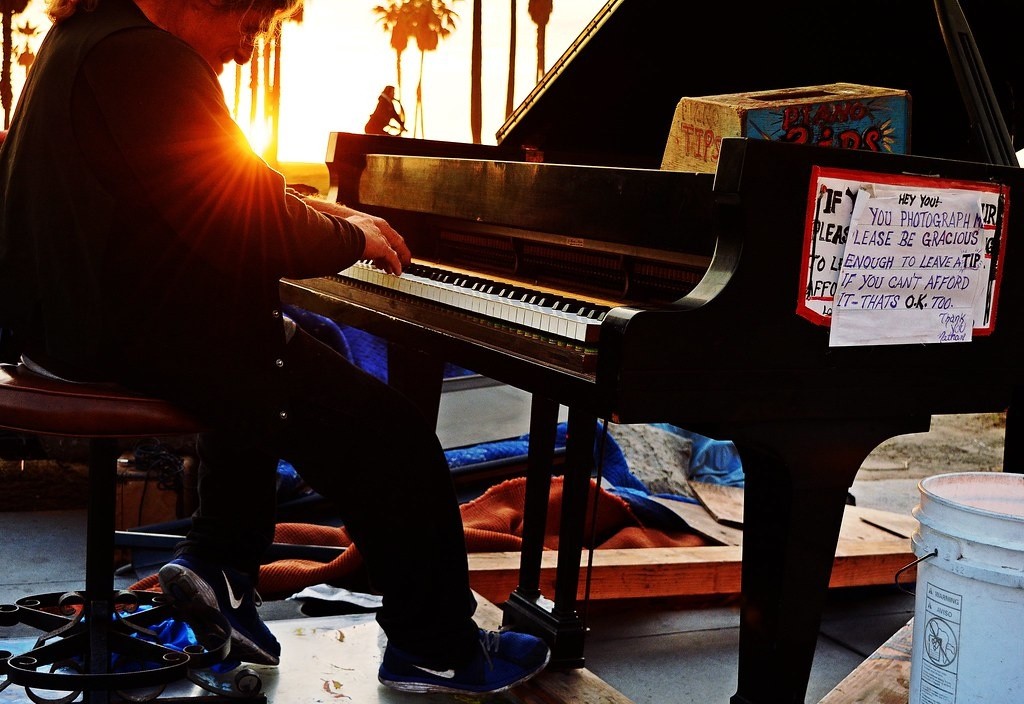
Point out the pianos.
[278,0,1024,704]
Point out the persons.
[1,1,553,702]
[363,86,408,136]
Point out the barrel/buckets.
[896,470,1024,704]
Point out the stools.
[0,363,267,704]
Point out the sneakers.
[378,626,551,697]
[158,556,280,665]
